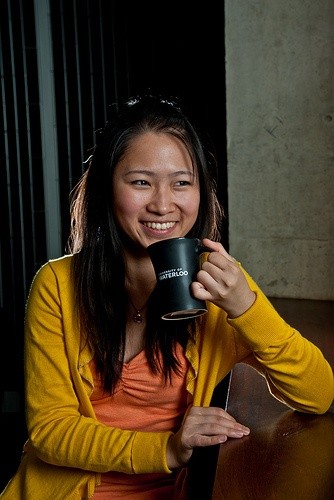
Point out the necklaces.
[127,294,147,324]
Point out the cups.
[146,238,215,322]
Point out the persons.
[0,99,334,500]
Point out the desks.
[211,295,334,500]
